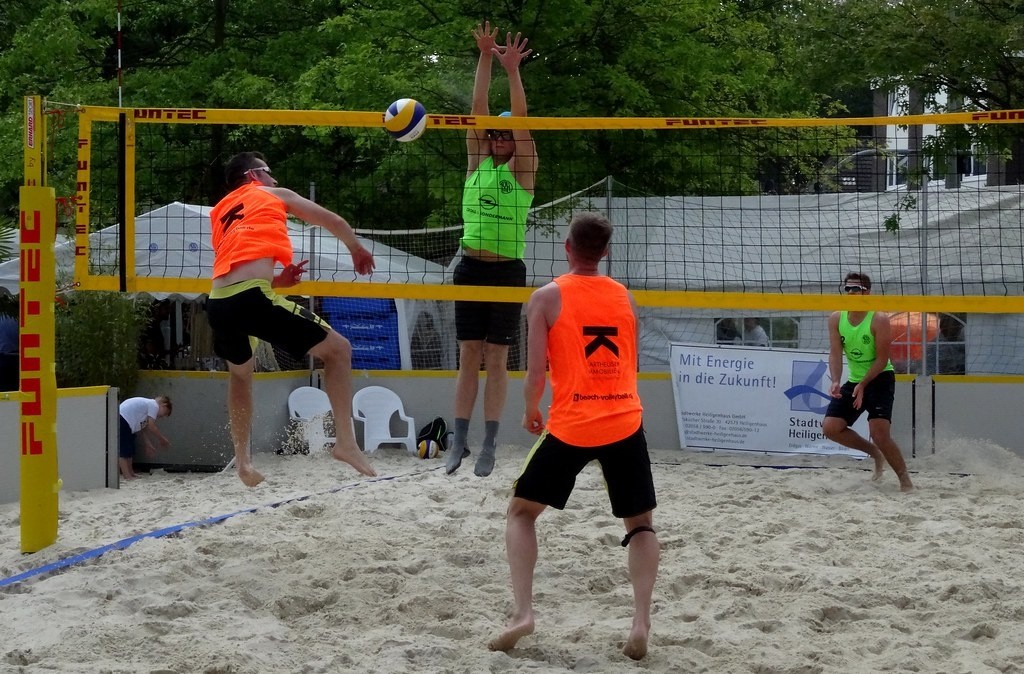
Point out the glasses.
[244,167,271,174]
[844,285,867,292]
[490,130,512,141]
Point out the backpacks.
[417,417,454,452]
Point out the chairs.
[289,385,335,454]
[353,384,418,455]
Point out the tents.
[445,184,1024,377]
[0,198,449,374]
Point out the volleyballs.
[380,99,429,141]
[417,440,439,459]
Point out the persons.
[118,394,173,481]
[205,150,378,488]
[716,313,771,348]
[442,18,539,479]
[922,314,967,374]
[139,297,214,370]
[822,271,913,493]
[487,212,661,662]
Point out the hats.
[499,111,511,117]
